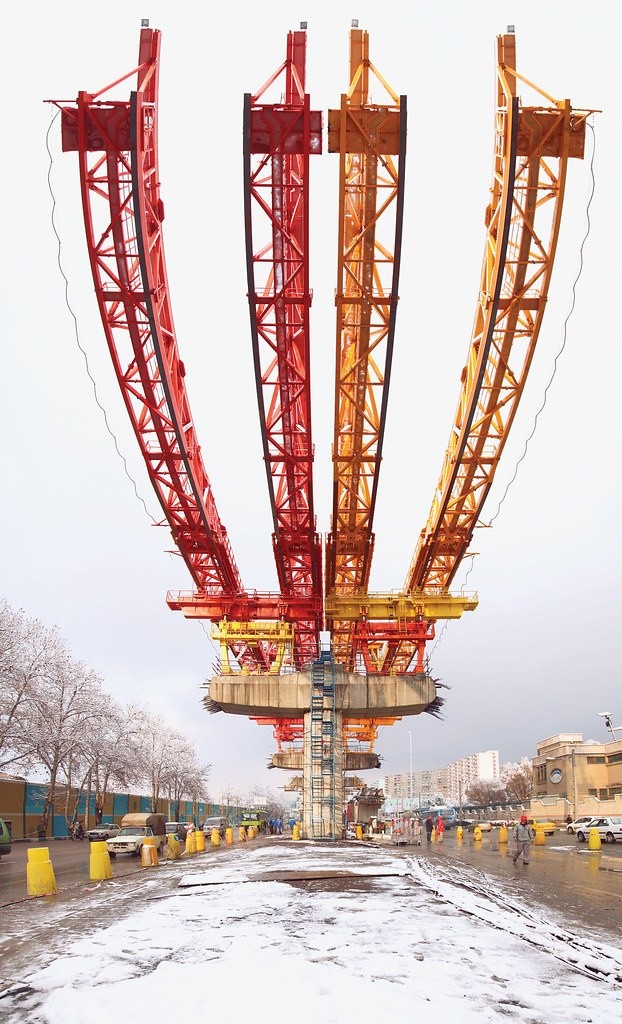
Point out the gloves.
[531,838,535,843]
[514,837,517,843]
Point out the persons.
[512,816,534,865]
[566,814,573,825]
[426,816,444,843]
[262,816,297,836]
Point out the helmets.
[374,815,377,819]
[370,815,374,819]
[521,816,527,821]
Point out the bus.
[421,809,456,830]
[241,809,268,831]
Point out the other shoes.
[522,862,529,865]
[438,841,443,843]
[427,841,432,843]
[513,856,516,863]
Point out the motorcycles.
[71,827,85,841]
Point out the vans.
[202,816,229,838]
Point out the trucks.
[106,813,167,858]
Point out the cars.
[86,823,121,841]
[567,816,608,834]
[527,818,556,836]
[182,822,194,831]
[165,822,187,842]
[466,820,492,832]
[576,817,622,844]
